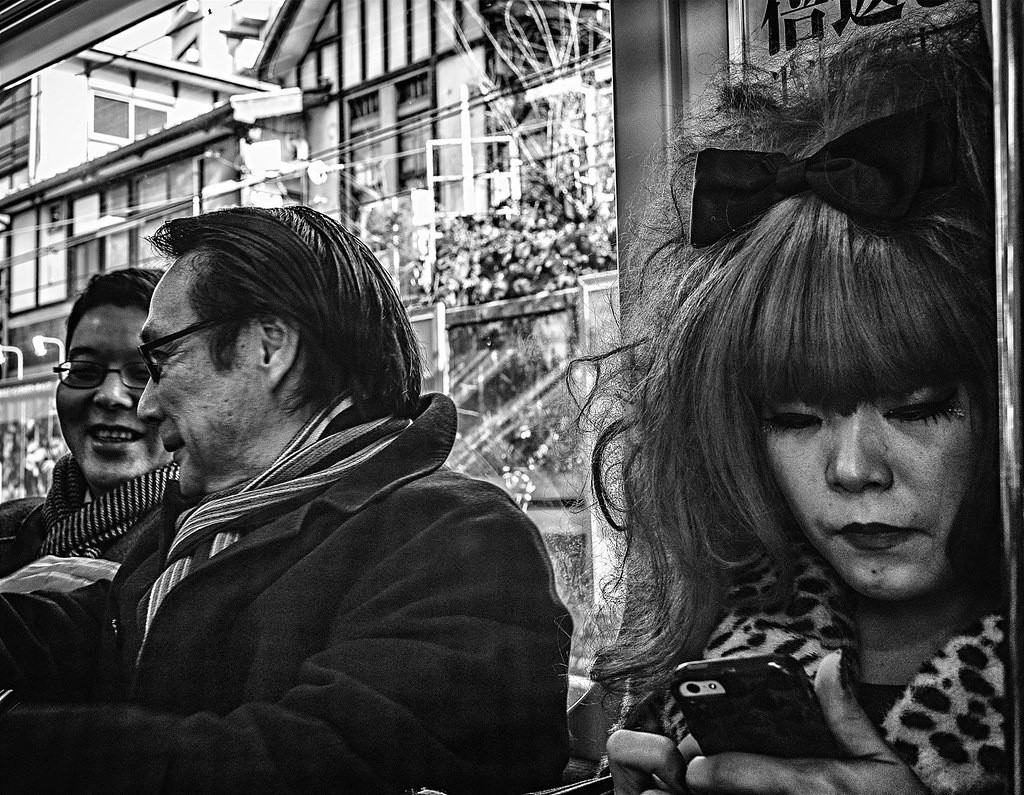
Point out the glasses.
[137,312,272,382]
[52,359,149,389]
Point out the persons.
[0,269,180,701]
[1,206,575,795]
[591,6,1012,795]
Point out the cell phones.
[670,655,840,761]
